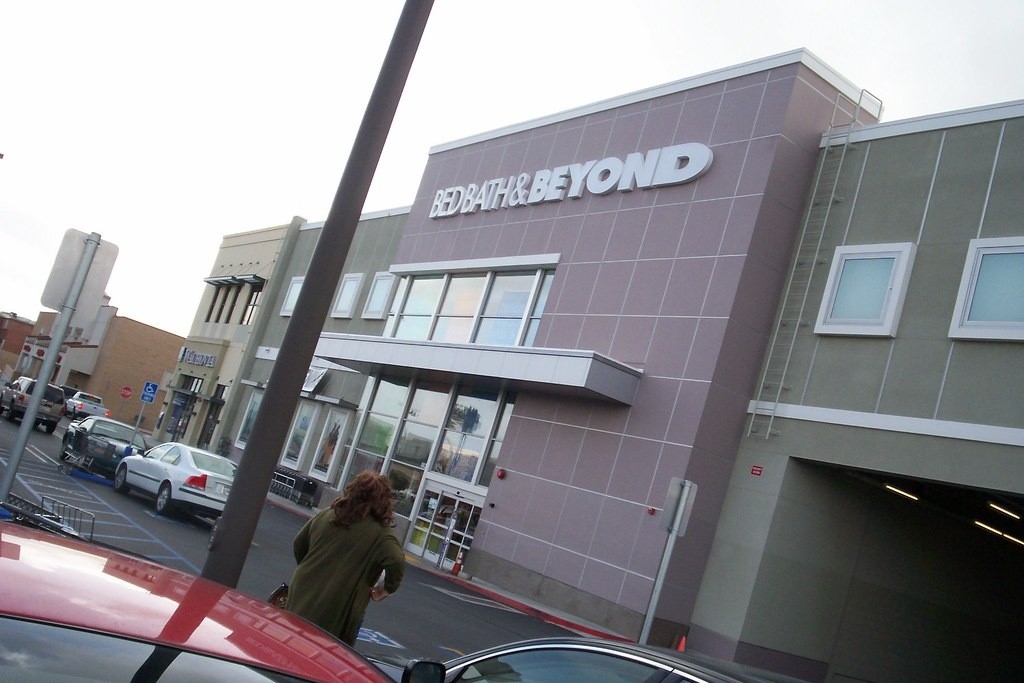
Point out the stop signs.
[121,386,132,399]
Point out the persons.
[286,470,405,648]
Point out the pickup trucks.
[59,384,110,420]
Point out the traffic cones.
[447,549,465,577]
[677,636,686,652]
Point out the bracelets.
[384,591,391,597]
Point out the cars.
[115,442,239,515]
[60,415,147,481]
[0,520,400,683]
[365,637,812,683]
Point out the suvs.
[0,376,67,434]
